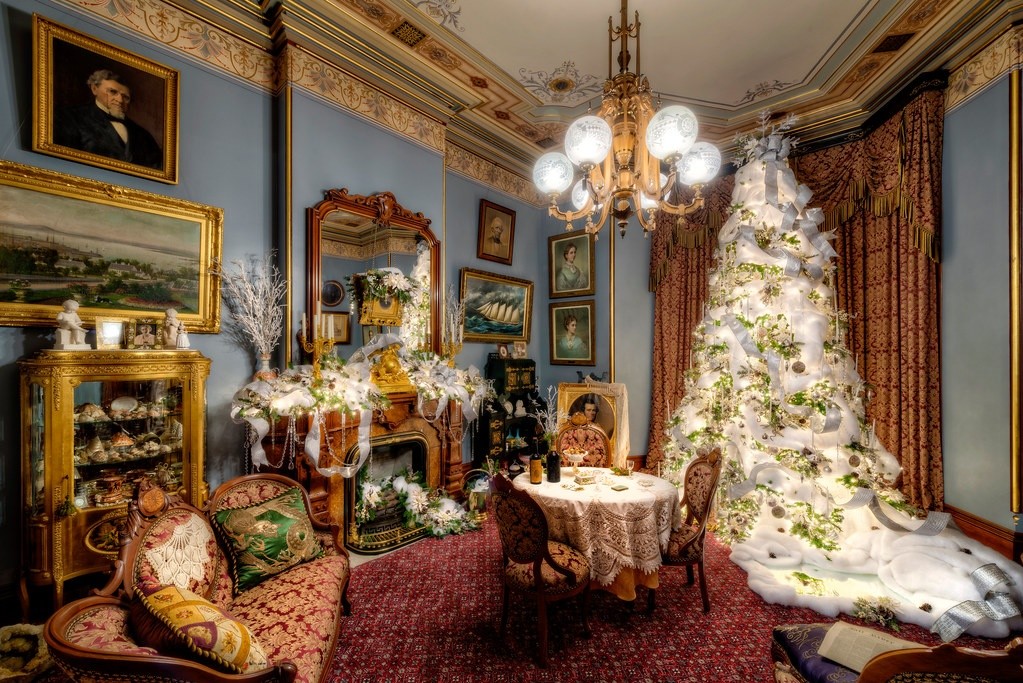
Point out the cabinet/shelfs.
[15,349,208,617]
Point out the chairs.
[487,470,593,668]
[553,412,612,469]
[624,445,722,622]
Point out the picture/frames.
[458,266,534,346]
[548,300,597,367]
[557,381,625,467]
[0,157,223,336]
[548,227,596,300]
[475,199,518,266]
[94,315,131,351]
[126,316,166,351]
[29,10,181,186]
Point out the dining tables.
[513,466,682,616]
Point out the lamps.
[531,0,723,241]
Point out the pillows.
[130,583,271,677]
[209,486,327,595]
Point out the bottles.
[547,434,560,483]
[530,436,543,485]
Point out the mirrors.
[304,187,441,374]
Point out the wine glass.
[627,460,635,479]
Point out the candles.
[427,313,464,344]
[302,301,334,341]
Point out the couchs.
[771,621,1023,683]
[44,474,354,683]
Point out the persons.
[56,299,90,344]
[136,324,157,345]
[174,320,191,349]
[162,308,180,346]
